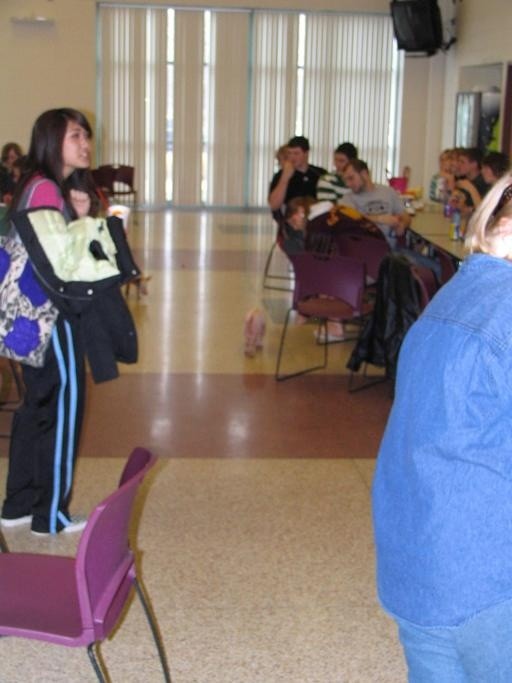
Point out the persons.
[0,107,132,538]
[370,168,510,681]
[1,141,23,200]
[3,154,33,206]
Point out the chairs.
[0,440,173,683]
[258,207,435,396]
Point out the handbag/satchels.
[0,236,59,368]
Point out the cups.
[107,203,130,231]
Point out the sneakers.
[1,514,87,536]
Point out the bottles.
[443,189,453,218]
[448,207,462,240]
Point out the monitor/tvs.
[390,0,444,57]
